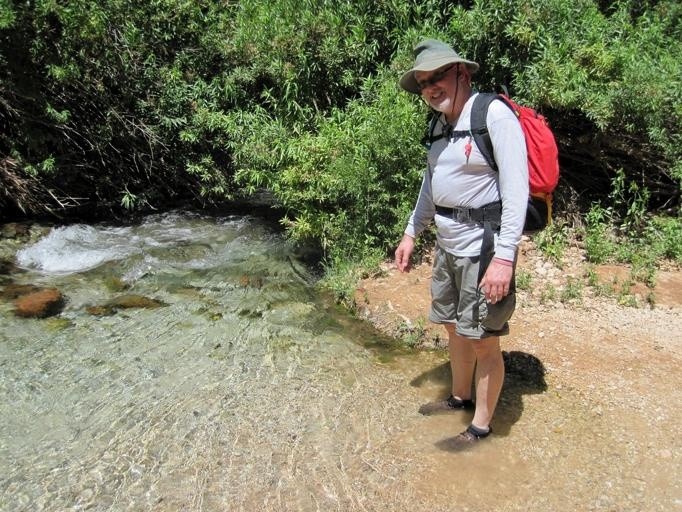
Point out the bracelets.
[490,257,513,267]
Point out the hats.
[399,38,479,94]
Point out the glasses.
[417,66,452,91]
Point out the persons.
[394,37,532,449]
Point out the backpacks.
[421,93,560,231]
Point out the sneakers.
[423,394,473,415]
[449,424,492,449]
